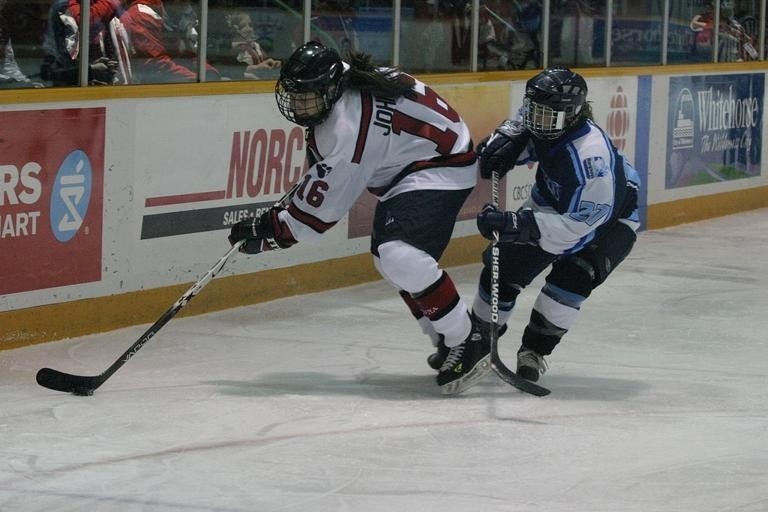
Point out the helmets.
[521,66,587,141]
[274,41,342,127]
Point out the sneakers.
[516,346,543,384]
[427,308,506,387]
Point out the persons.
[206,4,292,79]
[118,2,222,82]
[229,40,492,386]
[415,4,503,69]
[472,67,641,382]
[690,6,749,62]
[2,3,52,82]
[66,2,118,84]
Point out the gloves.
[476,203,540,243]
[227,207,298,254]
[476,120,529,178]
